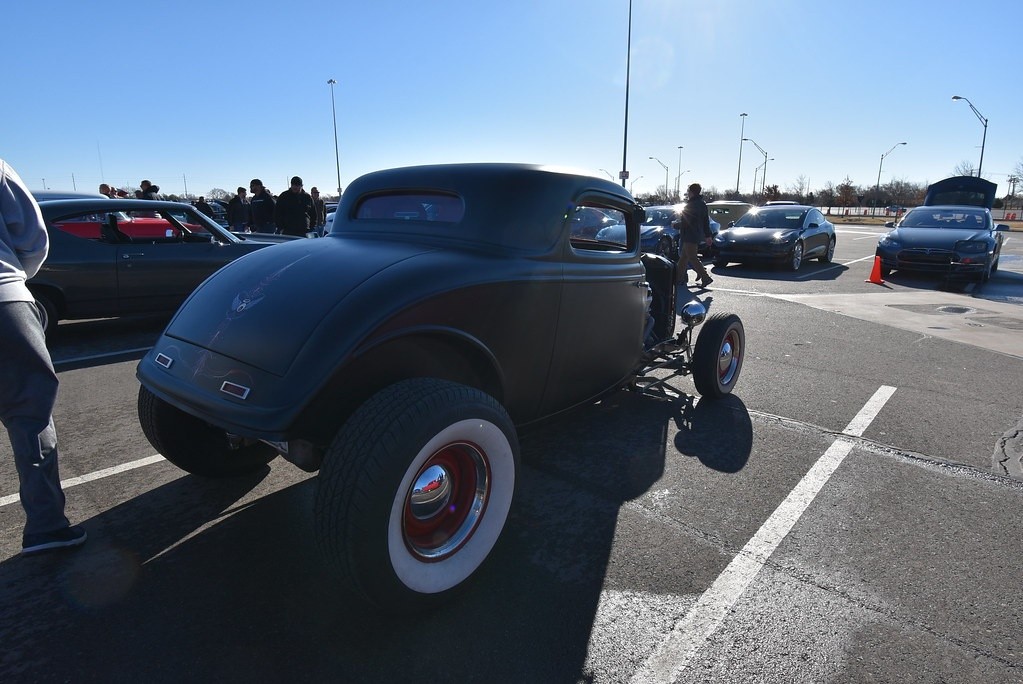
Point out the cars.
[24,186,343,346]
[764,201,801,207]
[705,199,754,230]
[594,203,722,261]
[568,207,618,238]
[710,205,838,273]
[874,175,1005,284]
[885,205,906,213]
[134,164,745,616]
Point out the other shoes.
[695,271,707,281]
[699,278,713,288]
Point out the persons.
[165,195,216,225]
[226,175,327,239]
[0,159,88,554]
[99,180,163,219]
[670,184,714,288]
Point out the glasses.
[140,184,144,188]
[251,184,257,187]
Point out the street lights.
[753,158,775,193]
[674,170,690,194]
[951,96,987,177]
[678,146,683,195]
[737,113,748,191]
[872,143,908,219]
[648,157,668,197]
[326,79,341,201]
[742,138,767,196]
[599,168,643,193]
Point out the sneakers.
[22,525,86,552]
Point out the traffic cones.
[865,256,886,283]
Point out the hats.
[311,187,319,193]
[250,179,265,188]
[291,177,302,185]
